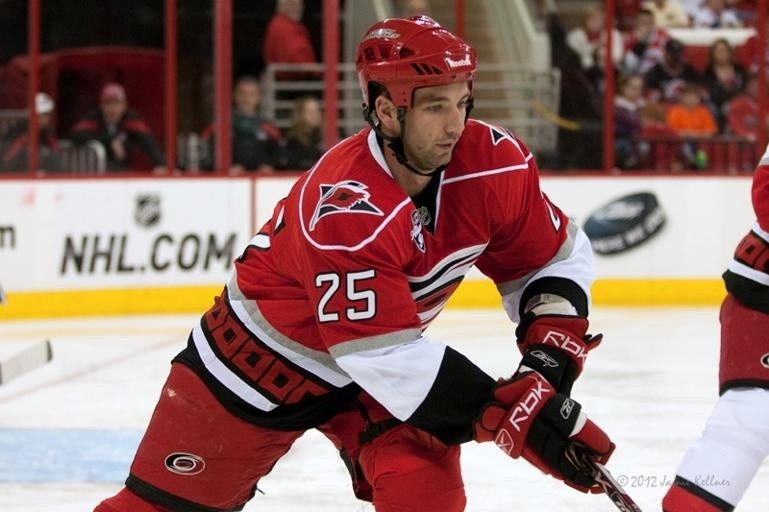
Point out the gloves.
[475,315,614,494]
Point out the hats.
[99,84,126,100]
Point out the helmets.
[356,14,478,109]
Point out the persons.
[562,0,769,171]
[92,16,616,512]
[1,81,166,175]
[176,2,325,174]
[661,144,769,512]
